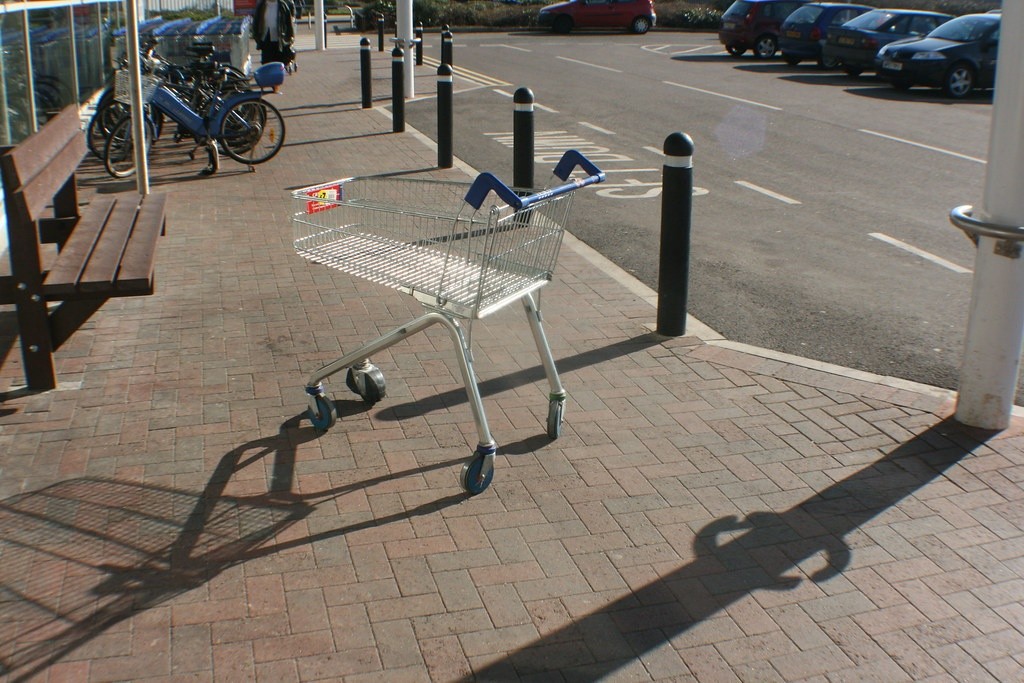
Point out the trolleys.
[1,20,110,146]
[110,15,252,78]
[282,150,608,497]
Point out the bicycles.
[89,39,286,179]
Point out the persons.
[281,0,296,34]
[247,0,298,96]
[293,0,307,20]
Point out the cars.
[719,1,805,59]
[877,10,1001,99]
[779,2,879,68]
[539,1,657,34]
[825,9,959,75]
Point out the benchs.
[1,102,170,389]
